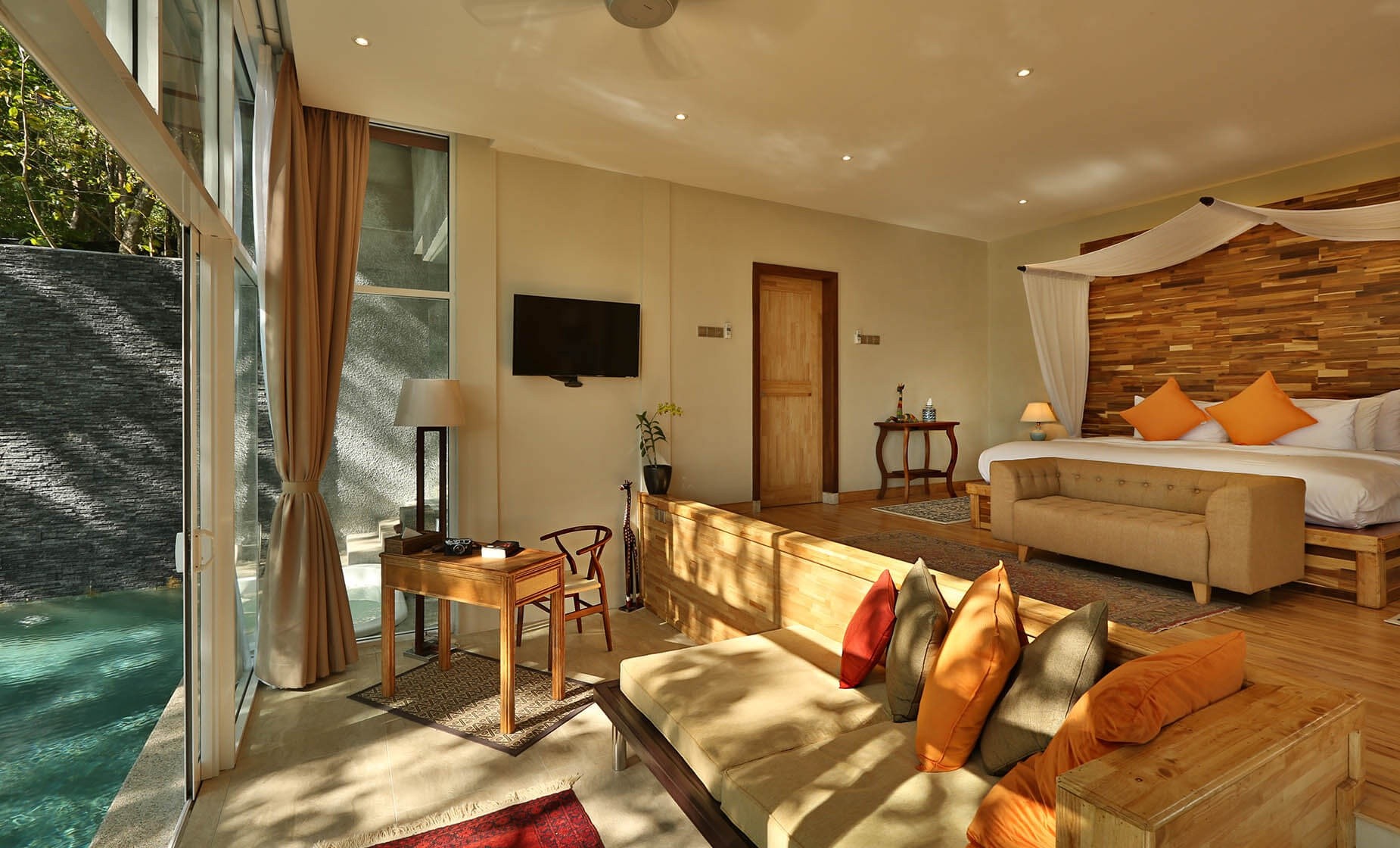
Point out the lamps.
[1018,401,1057,442]
[391,375,469,663]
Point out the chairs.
[514,524,615,673]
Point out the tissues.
[384,527,442,555]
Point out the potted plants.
[632,401,685,496]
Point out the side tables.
[966,480,991,529]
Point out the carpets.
[312,771,604,848]
[871,496,972,526]
[828,529,1244,635]
[347,647,598,758]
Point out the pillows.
[916,559,1021,774]
[1133,395,1230,443]
[965,629,1247,848]
[884,556,951,724]
[1119,375,1212,440]
[1270,397,1362,451]
[1205,369,1319,446]
[1364,388,1400,451]
[978,599,1109,776]
[1291,396,1388,451]
[838,569,897,689]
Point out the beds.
[965,369,1400,612]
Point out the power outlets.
[861,334,880,345]
[697,326,724,339]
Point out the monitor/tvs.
[512,294,640,378]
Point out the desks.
[873,420,961,504]
[379,535,569,735]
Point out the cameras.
[444,538,473,555]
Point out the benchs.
[593,545,1370,848]
[988,457,1307,605]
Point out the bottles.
[922,398,936,422]
